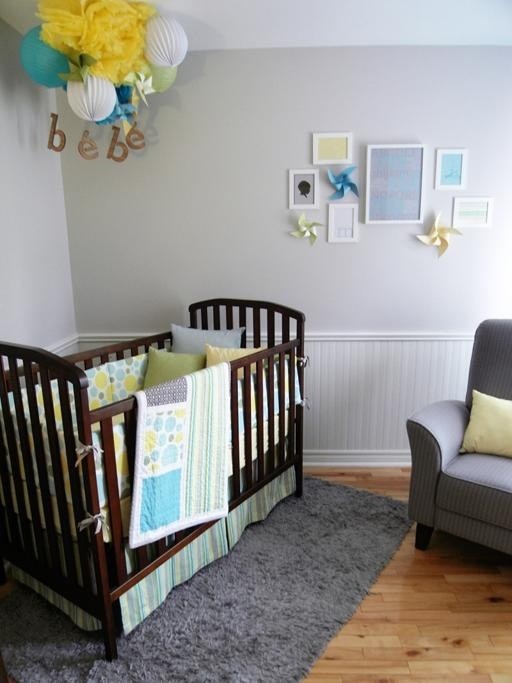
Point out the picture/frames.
[313,132,352,165]
[434,148,468,191]
[365,143,429,225]
[288,168,320,210]
[328,203,359,244]
[452,197,493,227]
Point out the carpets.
[0,476,416,683]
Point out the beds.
[0,298,306,662]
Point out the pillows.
[203,343,263,378]
[169,322,246,354]
[143,345,205,389]
[459,389,512,458]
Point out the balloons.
[151,67,176,93]
[19,25,70,87]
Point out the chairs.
[406,318,512,557]
